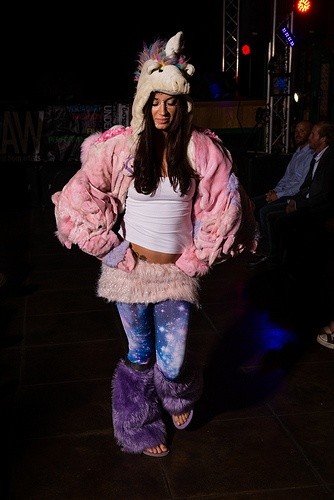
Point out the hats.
[130,31,196,134]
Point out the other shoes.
[317,327,334,349]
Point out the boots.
[154,364,198,429]
[111,362,170,457]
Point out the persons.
[243,120,334,265]
[52,31,257,456]
